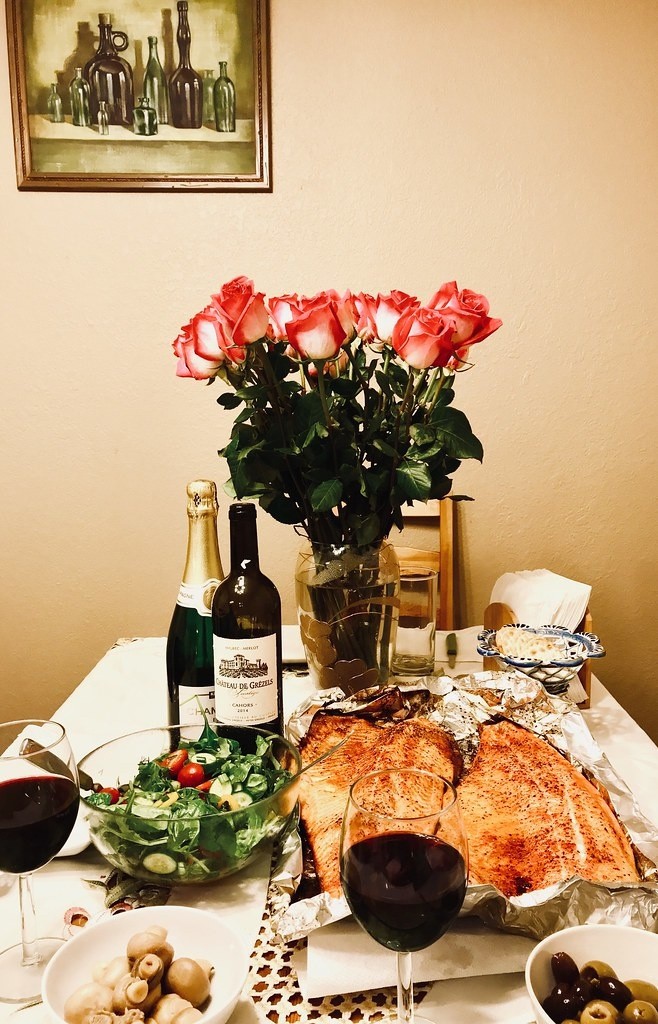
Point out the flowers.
[170,274,503,682]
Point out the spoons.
[18,736,106,795]
[274,727,355,793]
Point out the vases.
[295,536,401,696]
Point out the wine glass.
[0,718,84,1006]
[337,767,471,1024]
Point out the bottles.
[166,479,226,751]
[210,502,284,753]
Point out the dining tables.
[0,632,658,1023]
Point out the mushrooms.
[64,925,214,1024]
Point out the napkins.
[482,567,594,710]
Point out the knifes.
[444,632,459,670]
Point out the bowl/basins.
[523,921,658,1024]
[76,720,305,887]
[43,904,250,1024]
[476,621,605,696]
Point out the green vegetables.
[86,694,294,882]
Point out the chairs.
[385,497,453,633]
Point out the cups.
[392,566,441,674]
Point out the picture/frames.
[4,0,272,194]
